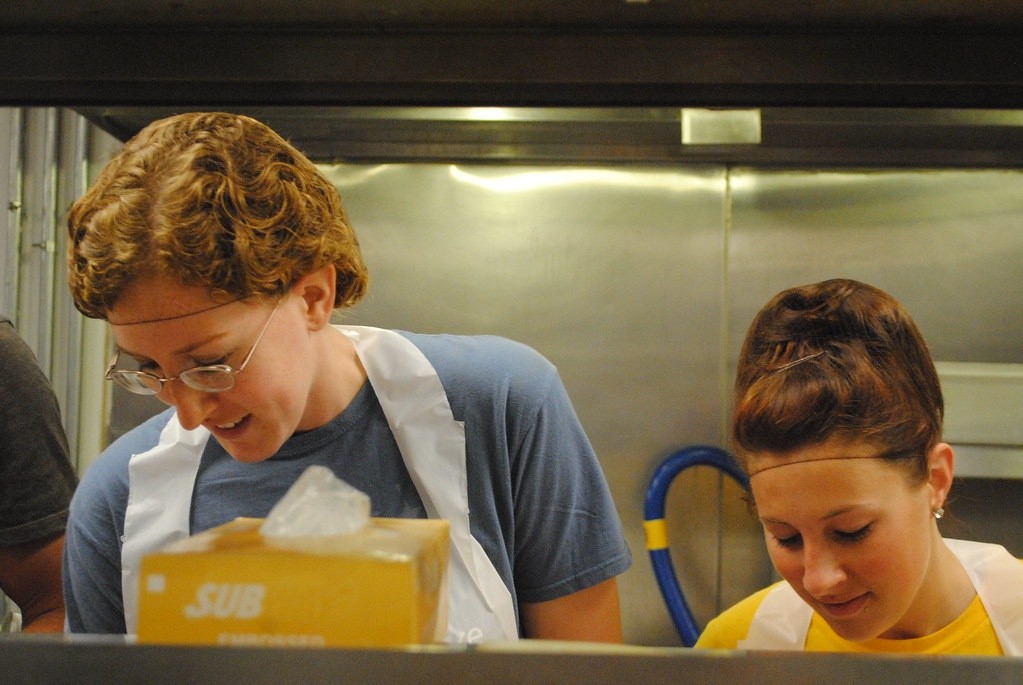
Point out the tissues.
[138,464,453,648]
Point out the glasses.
[101,282,301,398]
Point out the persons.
[0,314,79,634]
[689,276,1020,657]
[59,111,637,644]
